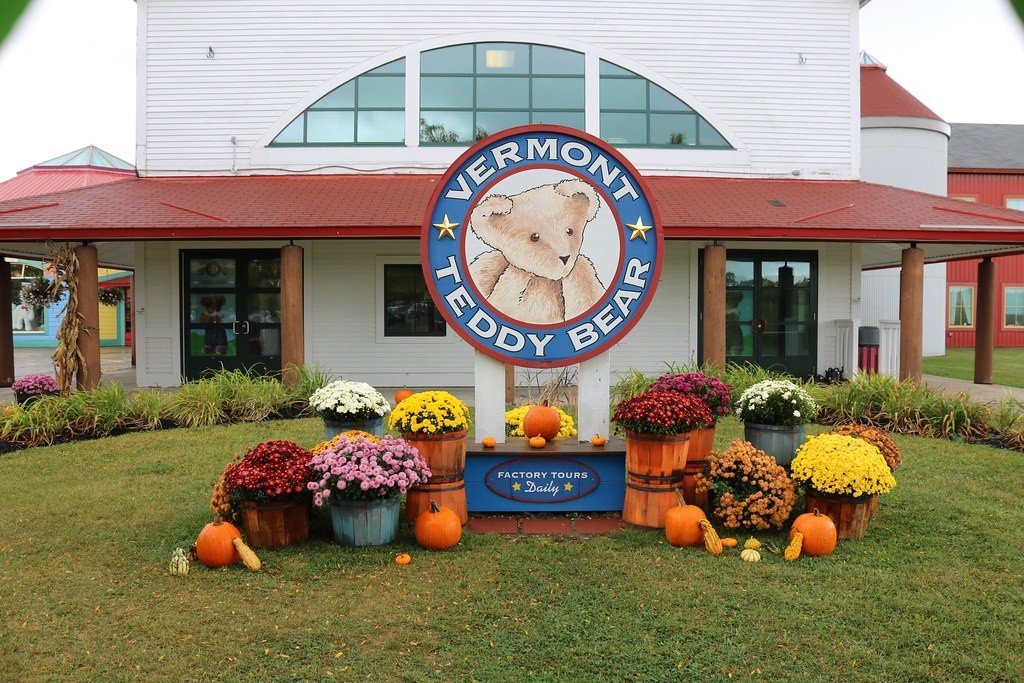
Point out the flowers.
[306,434,433,509]
[387,390,472,435]
[209,440,317,520]
[695,437,797,536]
[505,404,577,439]
[98,287,125,308]
[789,432,897,500]
[607,391,716,436]
[309,380,391,419]
[10,372,58,396]
[829,422,904,473]
[733,378,821,428]
[647,372,738,417]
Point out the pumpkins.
[395,385,413,404]
[697,519,722,555]
[169,548,190,576]
[523,399,560,442]
[784,532,803,561]
[232,536,261,572]
[414,500,462,550]
[592,434,605,446]
[187,546,198,562]
[721,538,738,547]
[529,434,546,448]
[196,513,244,568]
[481,437,496,447]
[741,547,760,562]
[395,553,410,565]
[790,508,837,556]
[664,488,708,547]
[743,536,761,550]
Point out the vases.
[238,500,309,546]
[401,428,469,483]
[15,392,37,410]
[805,487,875,539]
[328,498,400,547]
[679,426,716,488]
[623,429,691,531]
[324,418,383,440]
[743,421,804,468]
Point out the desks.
[464,442,627,512]
